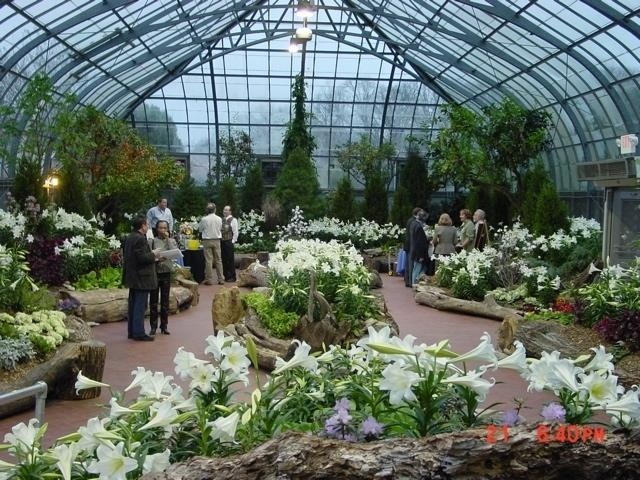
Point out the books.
[155,248,184,260]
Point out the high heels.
[149,328,157,335]
[160,327,170,336]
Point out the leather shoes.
[218,280,224,285]
[133,333,152,341]
[203,282,213,286]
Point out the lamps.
[286,36,299,58]
[45,170,60,198]
[294,24,315,40]
[292,0,317,21]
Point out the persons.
[455,210,475,251]
[146,195,173,238]
[147,220,178,335]
[433,213,458,273]
[473,209,487,252]
[410,210,429,284]
[198,202,225,286]
[404,207,421,287]
[221,205,239,282]
[122,213,161,341]
